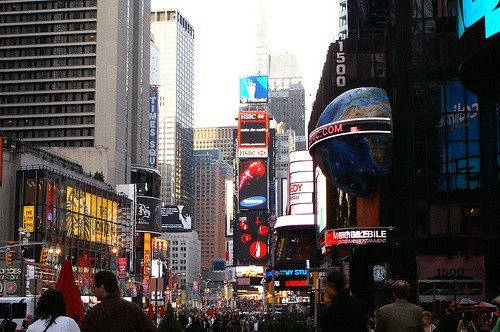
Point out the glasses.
[92,285,97,289]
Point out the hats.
[327,272,345,287]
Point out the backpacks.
[240,320,250,332]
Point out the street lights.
[111,242,120,277]
[49,248,61,287]
[199,277,211,308]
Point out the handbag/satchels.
[469,325,474,332]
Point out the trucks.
[0,297,38,332]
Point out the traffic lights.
[6,253,13,267]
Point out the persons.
[80,271,155,332]
[0,271,500,332]
